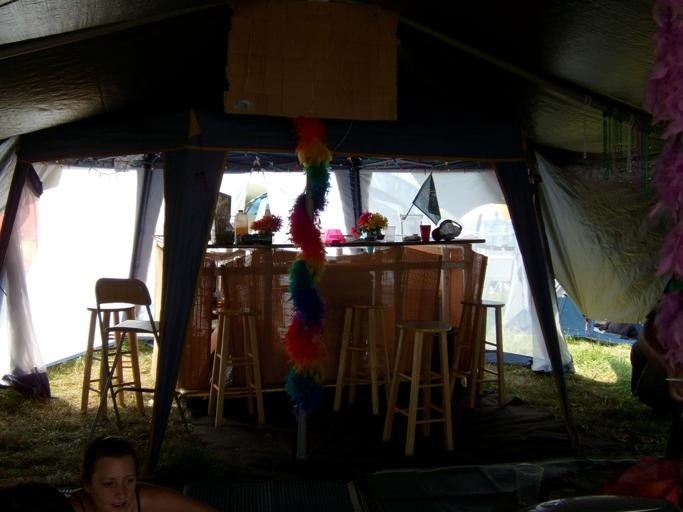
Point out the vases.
[366,227,385,240]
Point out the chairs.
[91,278,188,432]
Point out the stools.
[453,298,505,410]
[384,319,453,457]
[330,301,391,415]
[80,305,145,413]
[207,307,266,428]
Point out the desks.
[178,239,485,387]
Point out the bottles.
[233,209,248,244]
[262,200,273,244]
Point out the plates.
[534,494,667,512]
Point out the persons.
[604,269,683,511]
[0,482,74,512]
[68,434,217,512]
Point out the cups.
[400,213,422,240]
[385,225,395,241]
[512,462,545,506]
[419,224,431,243]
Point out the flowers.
[250,213,283,232]
[350,211,387,242]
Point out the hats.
[432,220,461,240]
